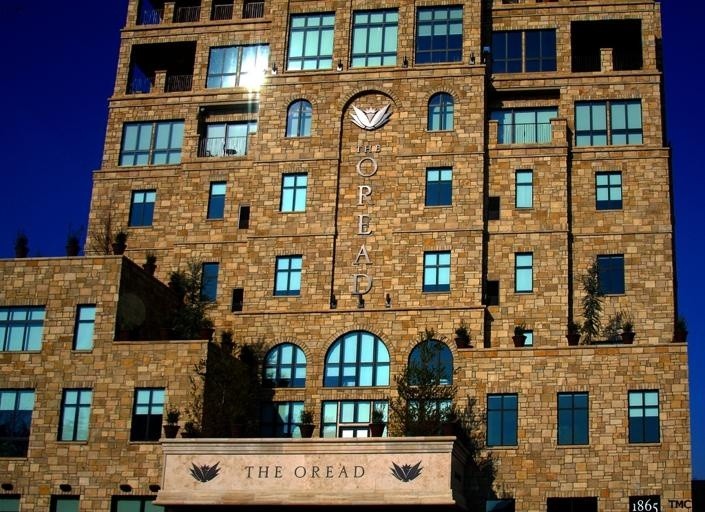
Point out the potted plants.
[673,317,689,342]
[141,254,157,276]
[510,325,527,349]
[117,317,259,366]
[620,321,636,343]
[368,404,458,436]
[166,271,181,292]
[565,320,583,346]
[63,235,81,256]
[295,408,317,438]
[161,406,249,439]
[453,326,471,349]
[110,230,128,255]
[13,233,31,258]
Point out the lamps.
[336,59,345,73]
[469,51,477,66]
[270,63,279,76]
[401,55,409,69]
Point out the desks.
[224,149,237,157]
